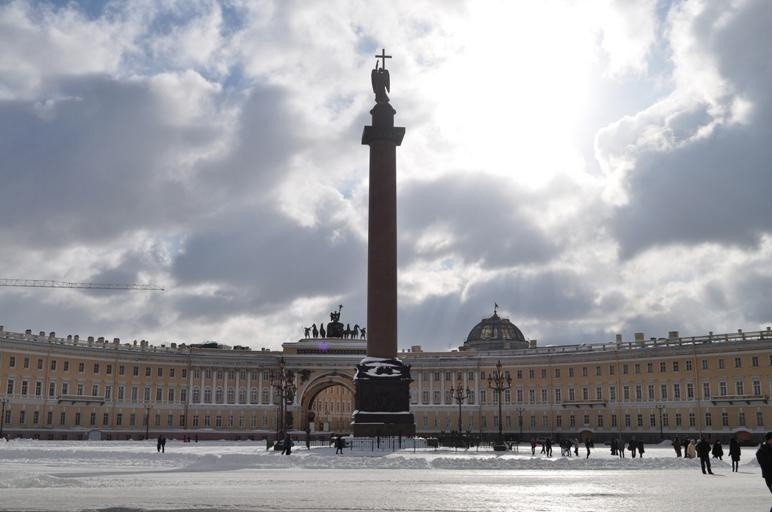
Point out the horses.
[320,323,326,338]
[311,323,318,338]
[337,324,360,339]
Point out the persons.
[531,438,555,457]
[375,68,390,103]
[560,438,579,456]
[585,438,590,459]
[282,436,294,455]
[359,327,366,339]
[672,437,723,475]
[157,434,166,453]
[728,437,741,472]
[756,432,772,512]
[610,438,645,458]
[305,327,311,339]
[336,435,344,454]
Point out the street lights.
[488,360,512,434]
[656,401,665,440]
[145,402,153,438]
[270,357,296,440]
[450,384,470,432]
